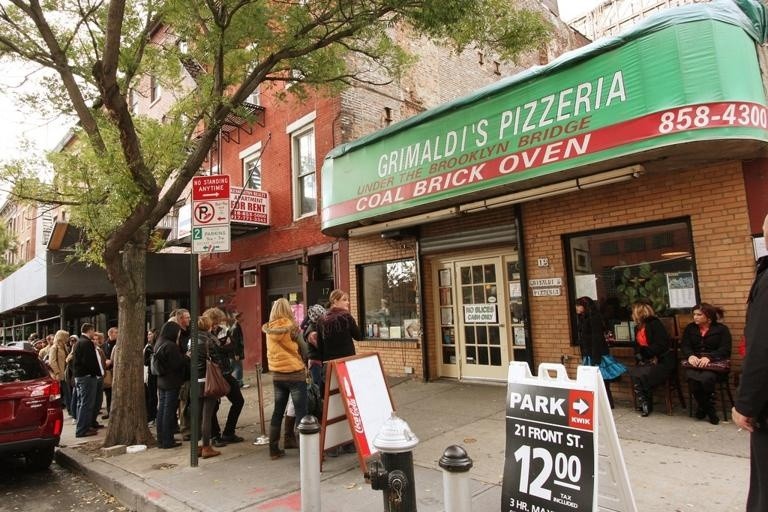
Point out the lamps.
[345,206,464,239]
[455,161,648,219]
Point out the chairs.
[630,334,687,418]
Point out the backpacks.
[150,340,171,376]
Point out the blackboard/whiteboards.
[337,352,393,462]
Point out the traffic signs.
[190,175,230,200]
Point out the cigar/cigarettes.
[738,428,743,432]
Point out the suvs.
[0,345,64,472]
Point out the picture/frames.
[572,245,592,274]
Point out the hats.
[307,304,326,324]
[634,298,653,306]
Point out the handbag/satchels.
[100,368,114,391]
[681,352,731,373]
[306,380,323,419]
[202,339,231,398]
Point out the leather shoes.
[221,434,243,444]
[212,435,225,447]
[63,404,109,424]
[147,414,202,449]
[76,427,97,437]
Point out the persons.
[731,213,768,512]
[574,297,629,410]
[631,298,677,417]
[680,304,731,427]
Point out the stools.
[685,372,736,423]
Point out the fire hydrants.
[362,408,423,512]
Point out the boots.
[201,447,220,459]
[197,445,204,456]
[634,383,653,416]
[284,416,299,449]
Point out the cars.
[4,340,30,349]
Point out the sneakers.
[695,408,706,420]
[270,449,285,460]
[708,408,720,425]
